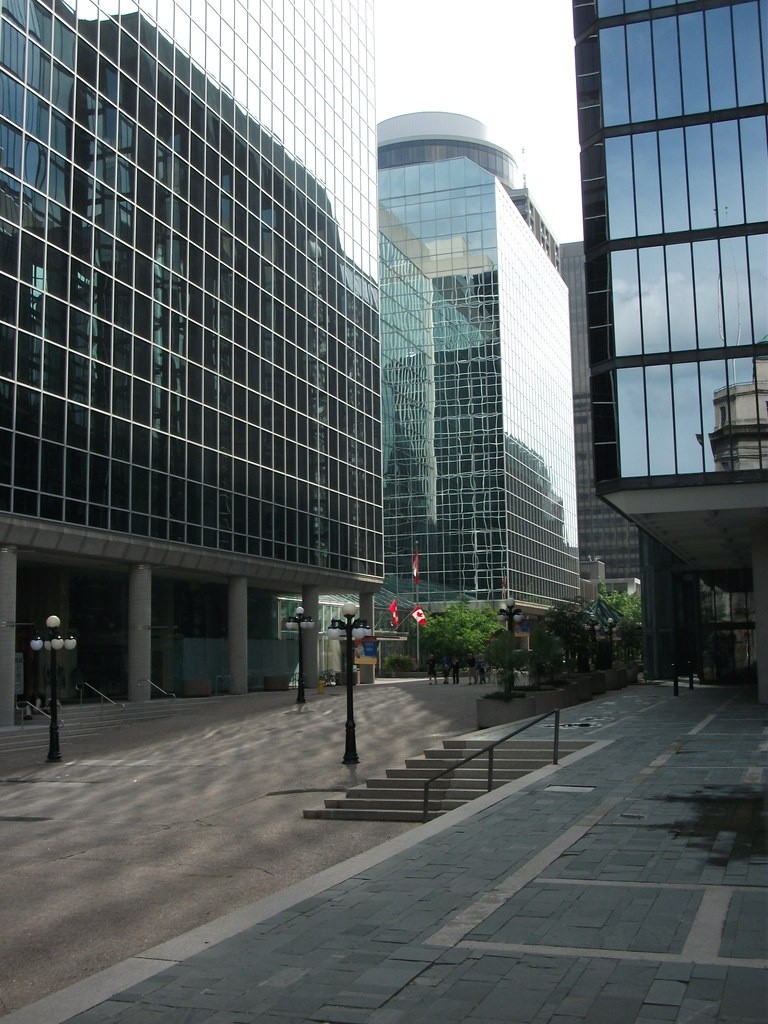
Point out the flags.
[411,553,419,585]
[389,601,400,625]
[411,607,427,626]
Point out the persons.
[441,657,491,685]
[425,654,438,685]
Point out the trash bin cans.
[354,656,378,684]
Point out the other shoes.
[429,683,432,685]
[443,681,449,684]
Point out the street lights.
[594,617,620,670]
[497,596,526,691]
[28,614,78,763]
[285,606,315,704]
[326,601,371,765]
